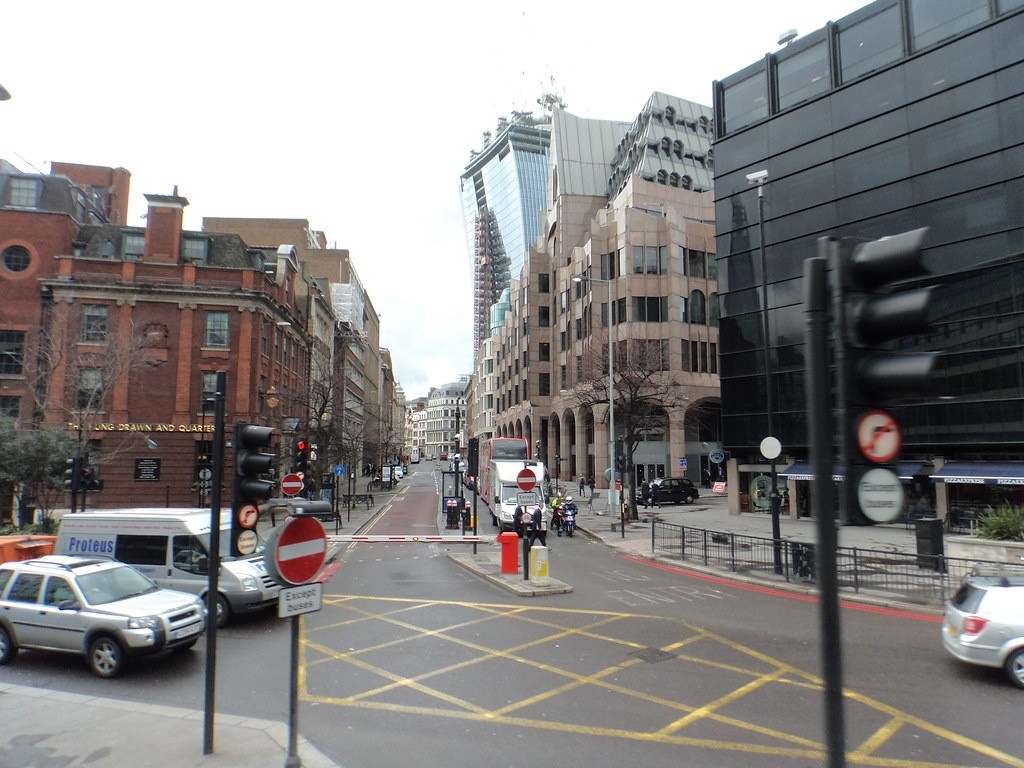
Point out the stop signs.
[273,518,328,583]
[517,469,536,492]
[280,473,302,495]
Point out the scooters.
[553,503,578,538]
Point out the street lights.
[196,397,217,507]
[571,274,620,532]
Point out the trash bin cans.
[790,543,815,582]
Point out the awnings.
[777,461,1024,486]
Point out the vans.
[53,506,286,632]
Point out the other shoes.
[558,531,563,536]
[550,527,553,529]
[548,548,552,551]
[574,526,578,530]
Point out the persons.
[515,504,524,537]
[641,478,650,509]
[579,479,586,497]
[550,492,578,537]
[588,476,595,494]
[528,502,552,552]
[651,479,662,509]
[704,468,712,489]
[718,466,726,482]
[366,463,370,476]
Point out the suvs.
[634,476,699,504]
[0,553,208,680]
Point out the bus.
[479,437,531,505]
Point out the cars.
[447,453,479,492]
[0,534,61,573]
[395,466,404,479]
[425,453,436,461]
[440,453,447,460]
[939,568,1024,688]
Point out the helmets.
[558,492,562,497]
[565,496,572,505]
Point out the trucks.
[490,459,548,537]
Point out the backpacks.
[580,478,584,486]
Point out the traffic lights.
[88,479,104,493]
[233,422,276,505]
[825,228,948,525]
[80,469,96,489]
[305,460,313,492]
[63,456,80,491]
[294,437,308,472]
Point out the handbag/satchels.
[536,523,546,531]
